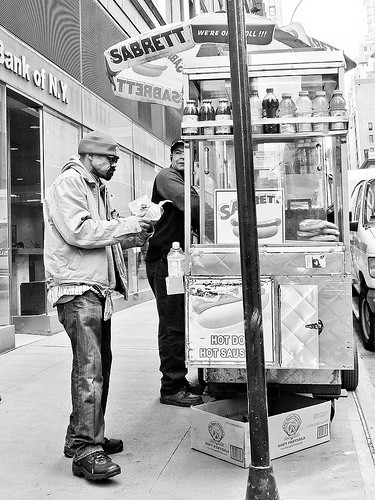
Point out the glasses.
[107,155,118,163]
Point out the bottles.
[283,139,317,174]
[183,88,347,137]
[167,241,186,277]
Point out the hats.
[78,130,119,158]
[171,139,196,154]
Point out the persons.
[41,130,164,480]
[17,242,24,249]
[145,137,215,408]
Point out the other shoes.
[72,452,121,480]
[160,390,204,407]
[64,437,123,458]
[185,382,203,395]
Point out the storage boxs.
[190,389,332,468]
